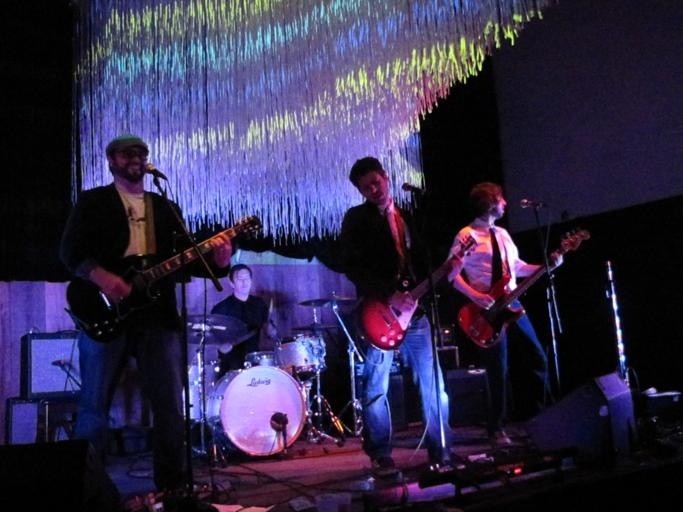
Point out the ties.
[384,207,400,253]
[488,228,503,288]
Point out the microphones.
[401,181,425,197]
[142,161,167,182]
[517,198,547,210]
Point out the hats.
[105,135,150,156]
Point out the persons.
[212,265,279,371]
[341,153,467,478]
[63,137,231,497]
[448,182,549,447]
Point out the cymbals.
[291,321,339,334]
[175,313,256,344]
[300,297,356,307]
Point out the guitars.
[458,231,590,349]
[351,234,478,352]
[66,214,262,343]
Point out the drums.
[206,366,307,456]
[244,351,275,366]
[275,333,326,371]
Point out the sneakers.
[489,430,511,449]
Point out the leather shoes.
[370,456,396,470]
